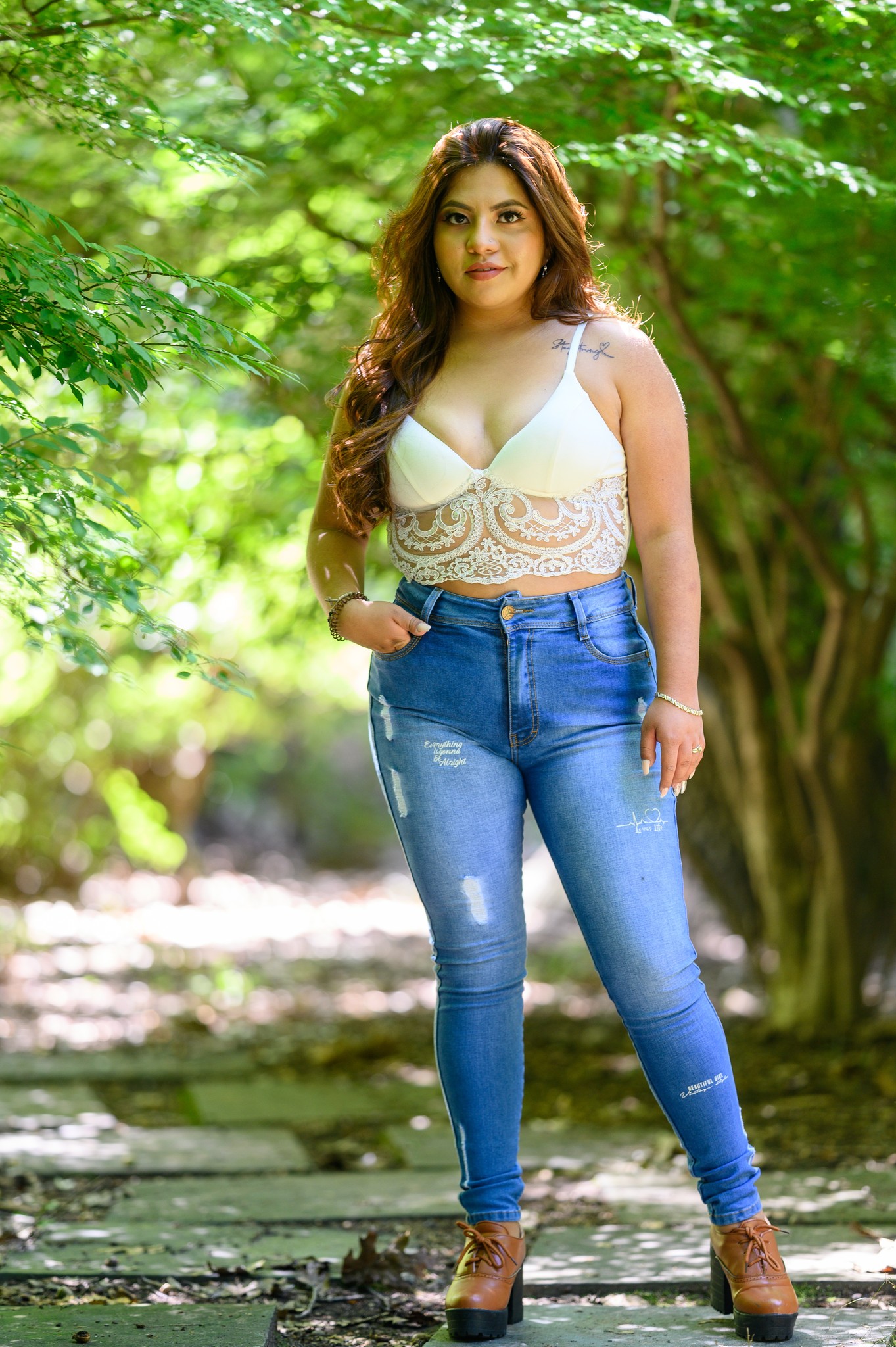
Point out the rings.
[691,745,703,754]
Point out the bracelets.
[324,591,370,642]
[654,691,703,718]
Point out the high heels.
[710,1215,800,1342]
[444,1221,526,1341]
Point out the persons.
[307,115,799,1343]
